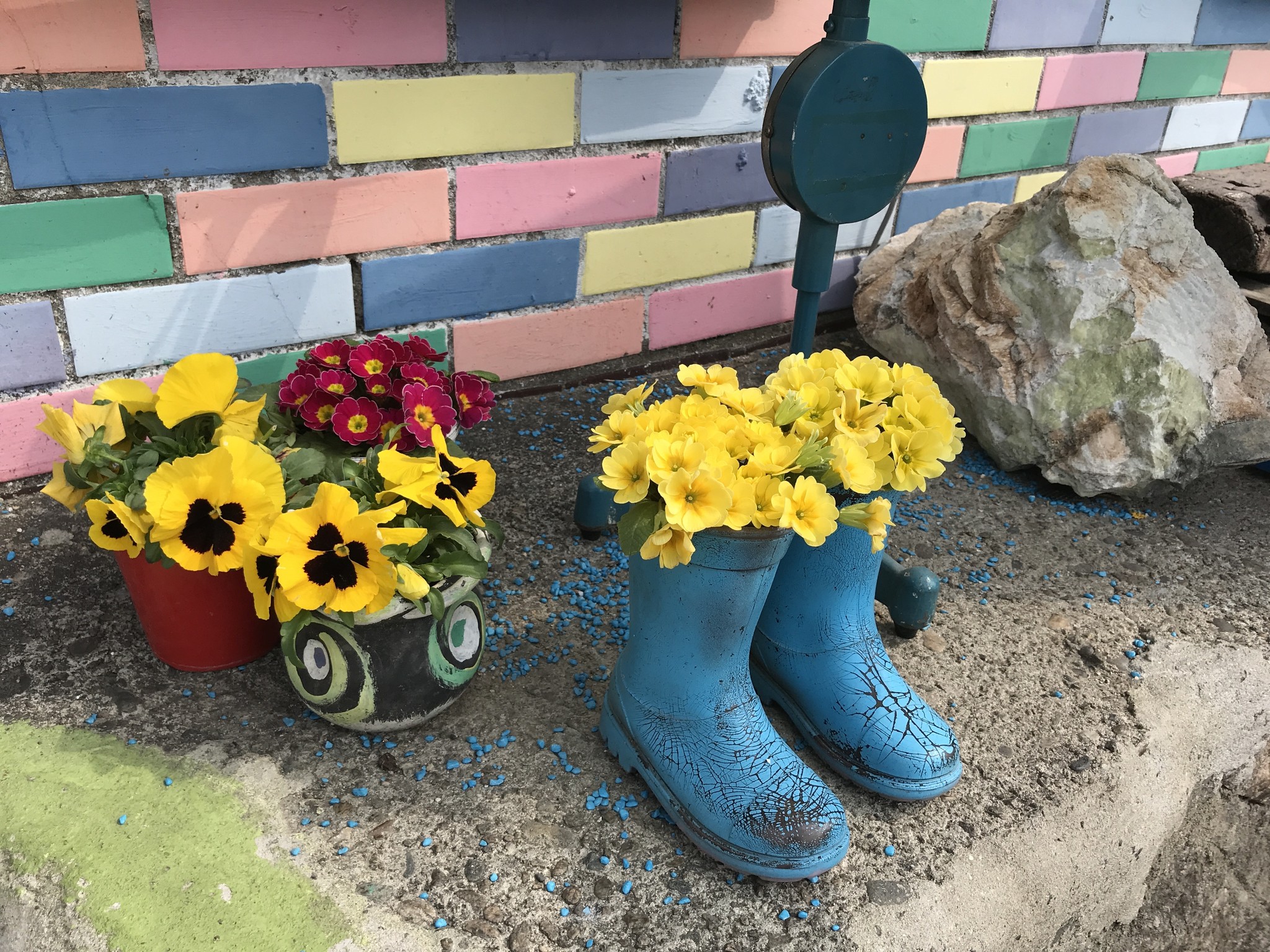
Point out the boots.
[748,485,970,803]
[596,485,855,884]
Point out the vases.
[106,534,277,672]
[284,526,488,734]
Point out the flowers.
[30,332,514,632]
[583,346,969,558]
